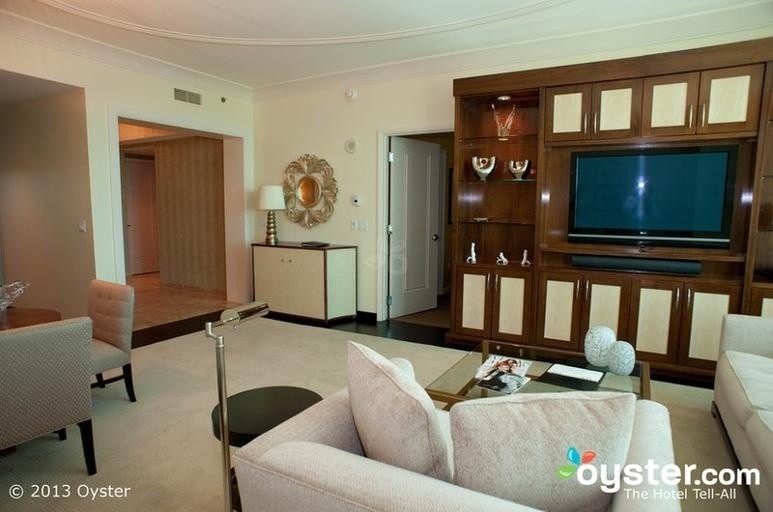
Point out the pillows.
[450,391,637,512]
[347,341,453,486]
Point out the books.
[301,240,329,248]
[474,353,533,395]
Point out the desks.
[0,309,61,457]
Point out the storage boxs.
[425,340,650,412]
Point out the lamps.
[206,302,268,512]
[256,185,286,245]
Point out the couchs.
[712,315,773,511]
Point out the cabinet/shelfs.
[742,286,773,317]
[451,69,545,268]
[741,38,773,289]
[545,56,643,147]
[536,272,631,357]
[252,245,357,328]
[627,278,742,377]
[641,38,765,143]
[456,267,532,352]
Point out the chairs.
[0,317,97,475]
[232,357,680,512]
[88,280,137,402]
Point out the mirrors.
[282,154,337,229]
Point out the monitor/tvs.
[568,145,738,249]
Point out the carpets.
[670,405,758,512]
